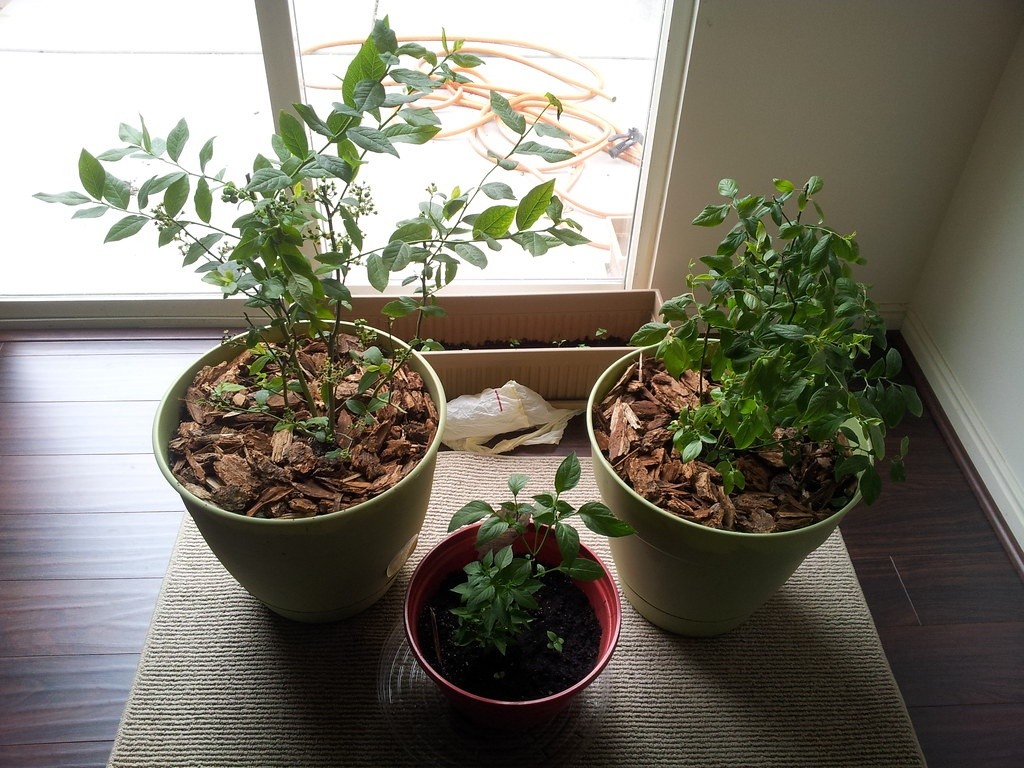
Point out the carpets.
[105,447,927,767]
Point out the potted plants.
[586,174,925,637]
[317,289,671,403]
[34,17,592,622]
[404,452,638,727]
